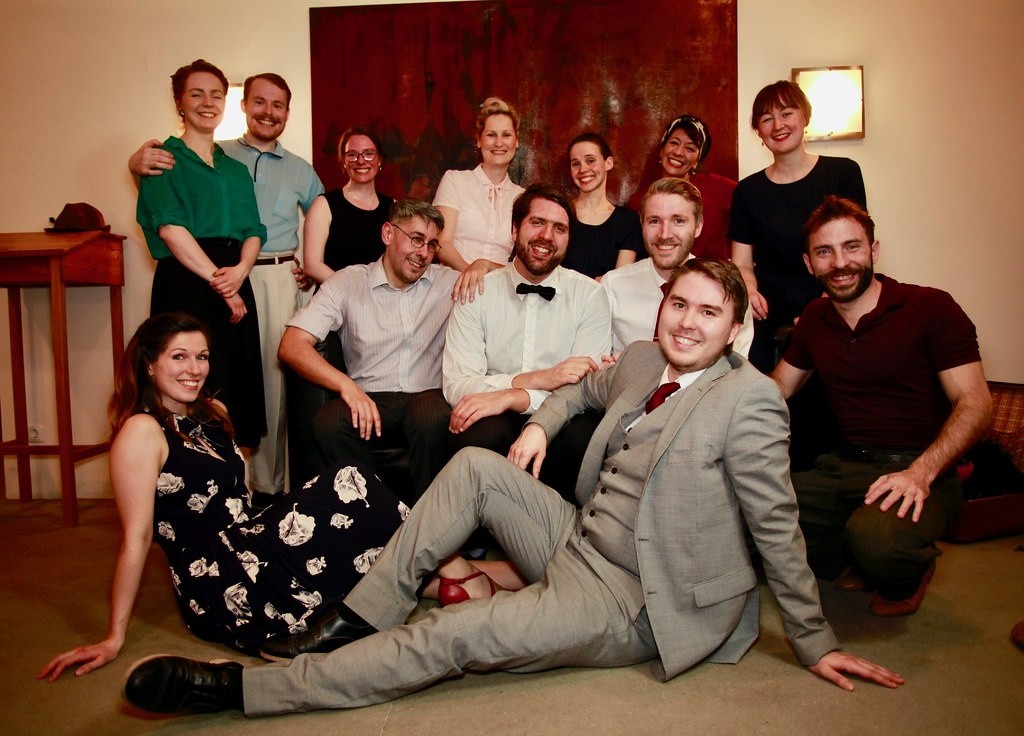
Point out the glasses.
[342,149,378,162]
[391,224,442,253]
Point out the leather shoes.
[258,610,367,663]
[120,655,247,715]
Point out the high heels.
[438,568,501,609]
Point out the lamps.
[791,63,866,143]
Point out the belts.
[253,256,294,266]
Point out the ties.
[654,283,671,344]
[644,380,681,414]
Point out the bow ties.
[516,282,556,301]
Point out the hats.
[44,202,111,232]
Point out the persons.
[442,182,612,502]
[430,98,525,305]
[558,132,642,283]
[36,310,525,684]
[129,73,325,495]
[730,80,868,377]
[303,127,398,374]
[136,59,269,487]
[277,198,505,501]
[773,196,995,618]
[628,114,738,261]
[123,258,905,717]
[599,178,753,369]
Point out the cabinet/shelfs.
[0,231,129,527]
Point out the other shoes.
[868,557,936,617]
[841,573,867,592]
